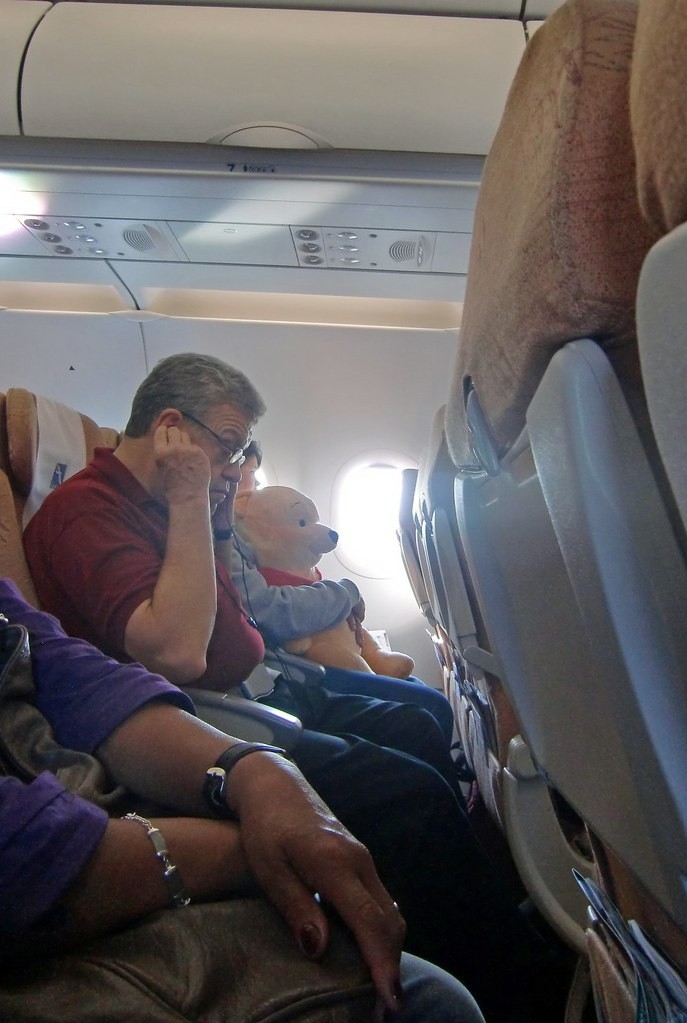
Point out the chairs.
[0,387,330,757]
[394,0,687,1023]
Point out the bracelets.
[120,812,190,909]
[202,741,298,819]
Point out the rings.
[392,902,399,912]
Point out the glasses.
[180,412,246,466]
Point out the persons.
[0,351,577,1023]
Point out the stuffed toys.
[233,486,415,680]
